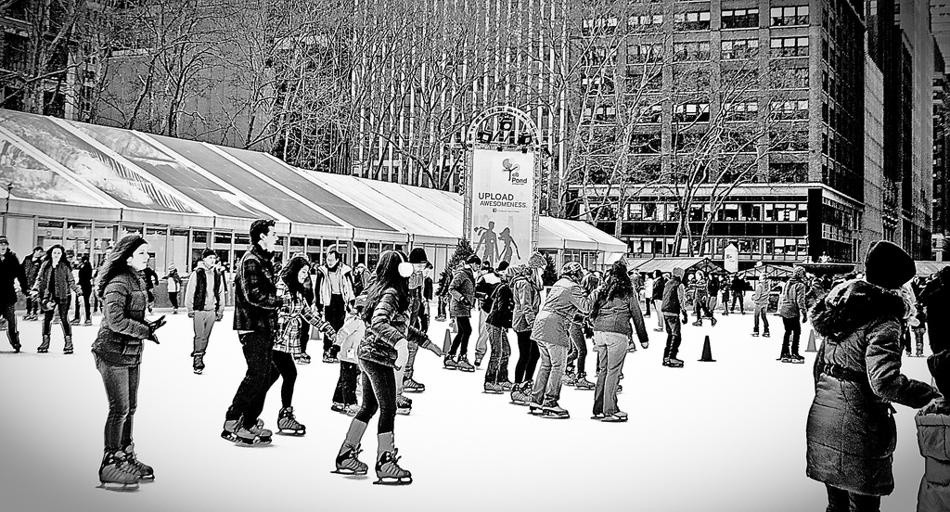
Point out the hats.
[864,239,916,291]
[202,247,216,259]
[168,265,176,272]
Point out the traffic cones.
[439,329,454,355]
[803,328,819,353]
[697,334,717,361]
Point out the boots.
[336,442,368,472]
[192,354,205,370]
[123,443,154,476]
[220,414,261,445]
[277,406,306,431]
[98,451,141,484]
[0,307,104,350]
[249,419,273,438]
[375,448,411,478]
